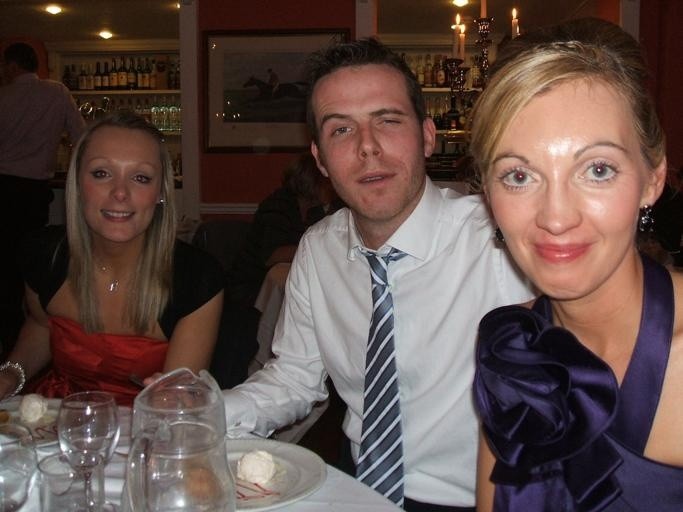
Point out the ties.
[357,246,409,506]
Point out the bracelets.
[0,360,26,403]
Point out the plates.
[226,437,327,512]
[0,409,60,447]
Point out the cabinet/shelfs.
[44,40,182,226]
[376,32,517,182]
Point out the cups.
[129,370,223,467]
[1,424,236,512]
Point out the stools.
[278,378,349,466]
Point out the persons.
[0,42,87,363]
[469,15,682,511]
[225,153,336,308]
[196,32,539,511]
[0,107,224,410]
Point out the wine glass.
[57,392,120,512]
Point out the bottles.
[59,52,181,130]
[174,152,182,190]
[397,48,474,133]
[439,140,460,173]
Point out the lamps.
[443,1,522,119]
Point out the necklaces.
[95,255,134,295]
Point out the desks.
[179,215,213,253]
[249,261,295,375]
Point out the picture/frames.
[201,27,350,157]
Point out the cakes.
[236,450,277,485]
[18,392,49,422]
